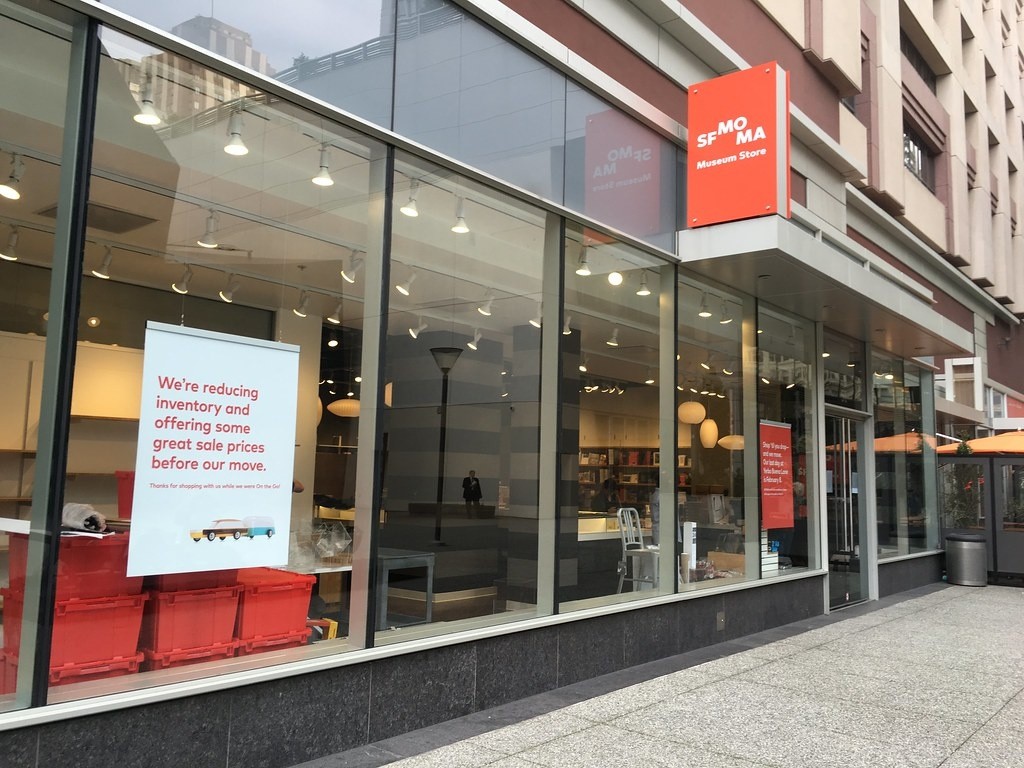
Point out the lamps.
[477,293,495,316]
[172,264,193,294]
[92,246,114,279]
[718,386,745,450]
[528,302,544,328]
[467,328,482,351]
[396,268,416,296]
[340,249,366,283]
[400,179,421,217]
[219,275,237,302]
[678,391,706,424]
[0,153,25,200]
[0,230,18,260]
[312,143,334,186]
[196,210,217,248]
[699,395,718,448]
[450,196,469,233]
[293,291,312,318]
[133,82,161,125]
[563,244,895,398]
[326,298,343,324]
[327,332,360,418]
[223,109,249,156]
[408,317,427,339]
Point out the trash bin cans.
[945,532,987,587]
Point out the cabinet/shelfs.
[66,414,139,480]
[577,445,693,529]
[314,444,390,609]
[0,447,37,554]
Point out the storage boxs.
[0,471,316,694]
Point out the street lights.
[428,346,465,541]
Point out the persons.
[590,478,619,513]
[650,472,682,555]
[293,479,304,492]
[462,471,482,505]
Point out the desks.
[267,563,352,635]
[375,547,436,632]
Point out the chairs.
[615,508,679,595]
